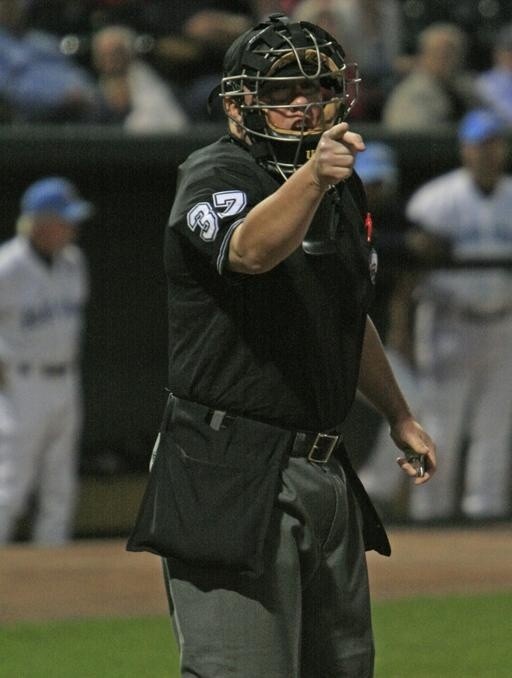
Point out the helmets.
[218,14,362,192]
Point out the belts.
[204,408,347,464]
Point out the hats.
[22,177,91,225]
[354,141,400,185]
[459,111,508,146]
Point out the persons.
[0,174,96,548]
[351,138,419,528]
[1,1,510,132]
[386,106,510,524]
[125,12,439,678]
